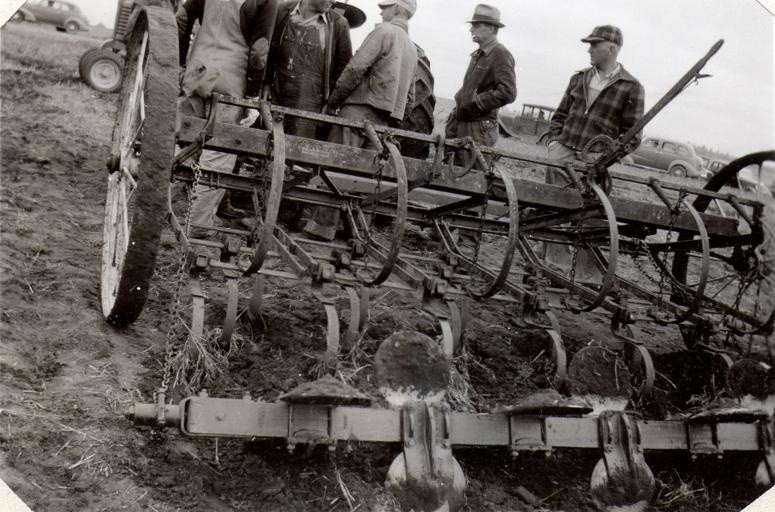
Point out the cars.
[620,136,774,198]
[9,0,91,35]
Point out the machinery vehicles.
[79,0,134,94]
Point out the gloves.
[240,93,262,129]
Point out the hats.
[466,3,504,29]
[582,25,625,44]
[379,1,418,17]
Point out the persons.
[542,25,645,281]
[434,4,516,259]
[171,1,420,258]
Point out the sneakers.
[216,192,247,220]
